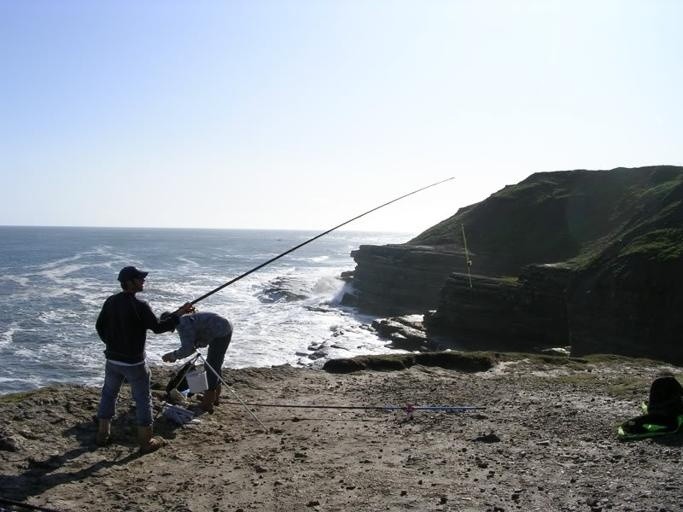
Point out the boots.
[97,418,110,446]
[137,425,163,454]
[192,390,216,415]
[197,385,221,405]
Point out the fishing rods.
[196,392,490,410]
[176,175,457,318]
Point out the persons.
[160,312,233,414]
[96,266,194,453]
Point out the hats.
[118,267,147,282]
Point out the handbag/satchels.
[618,377,683,447]
[166,363,197,398]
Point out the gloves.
[162,352,177,363]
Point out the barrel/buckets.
[186,369,209,394]
